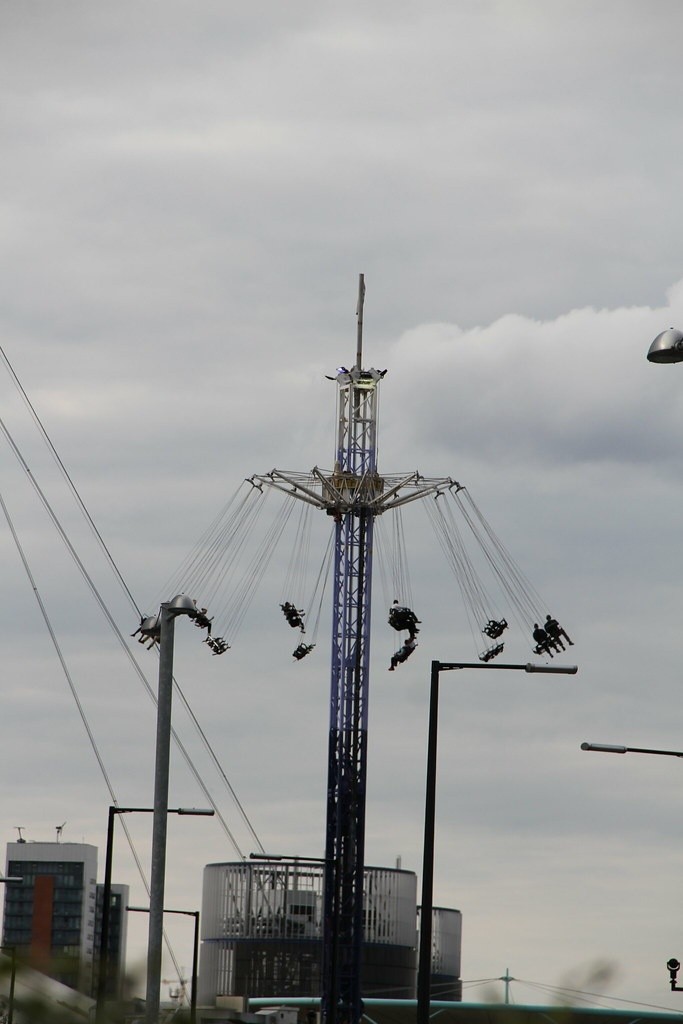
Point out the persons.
[188,599,215,636]
[131,614,161,650]
[280,602,306,633]
[388,638,415,671]
[390,600,422,639]
[544,615,574,651]
[533,624,560,658]
[202,636,231,655]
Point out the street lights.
[92,804,214,1024]
[125,906,200,1024]
[413,657,580,1023]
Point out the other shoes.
[563,647,565,650]
[556,650,560,652]
[569,643,574,645]
[550,655,553,657]
